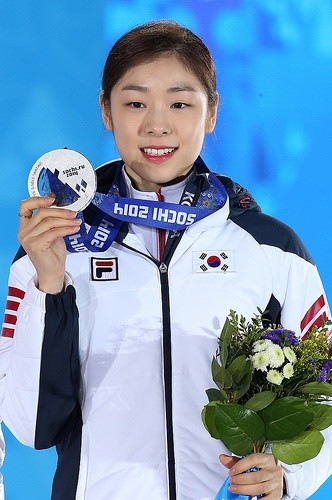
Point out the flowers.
[201,306,332,498]
